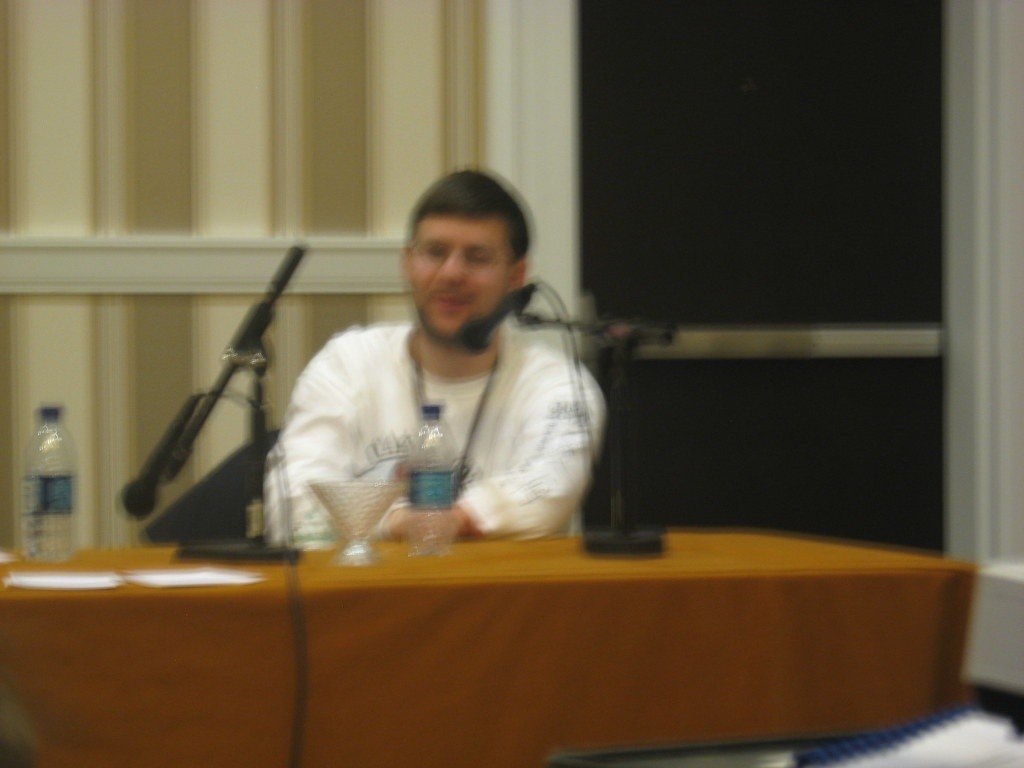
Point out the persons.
[263,171,604,556]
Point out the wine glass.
[308,478,406,570]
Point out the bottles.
[406,404,456,563]
[19,407,80,565]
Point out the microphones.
[120,393,203,518]
[454,285,538,355]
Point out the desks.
[1,514,978,768]
